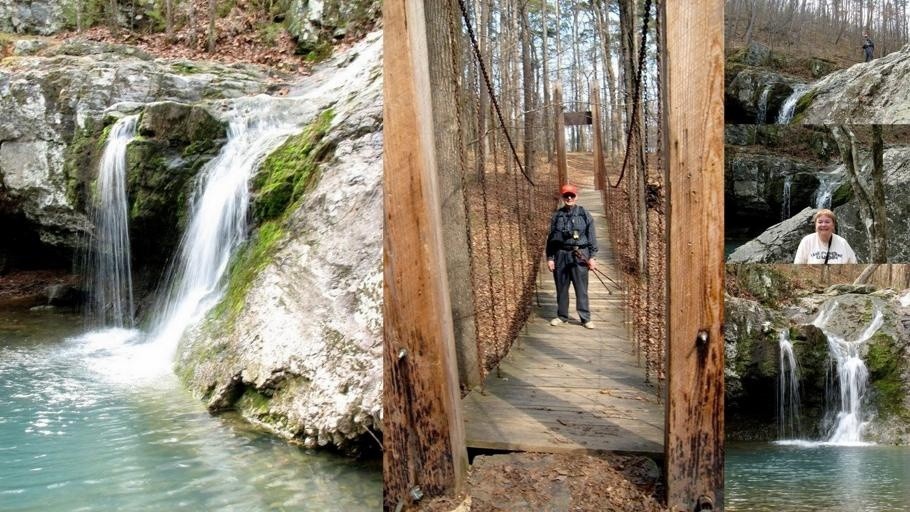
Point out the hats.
[561,185,576,195]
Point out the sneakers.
[581,320,594,329]
[551,318,568,326]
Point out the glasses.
[563,193,575,198]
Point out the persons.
[793,207,859,264]
[544,184,600,330]
[861,33,875,63]
